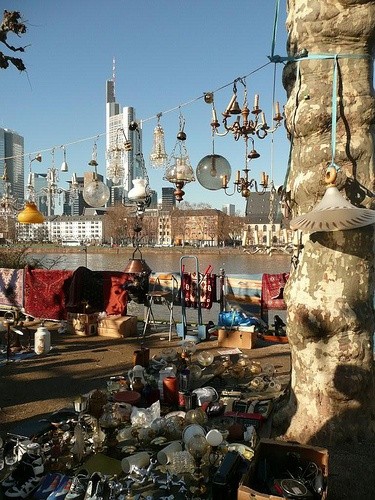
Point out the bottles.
[35,327,51,355]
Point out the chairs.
[143,273,177,342]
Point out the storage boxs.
[67,312,137,338]
[218,328,259,349]
[238,438,329,500]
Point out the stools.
[114,391,147,409]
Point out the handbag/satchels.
[218,311,245,332]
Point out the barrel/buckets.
[120,451,150,473]
[157,442,183,464]
[182,425,206,455]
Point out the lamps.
[188,429,223,500]
[290,168,375,232]
[1,77,283,222]
[124,219,152,273]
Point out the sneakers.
[33,471,63,498]
[65,469,90,500]
[4,461,47,498]
[47,474,75,500]
[2,453,45,487]
[84,472,105,500]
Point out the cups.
[183,392,197,410]
